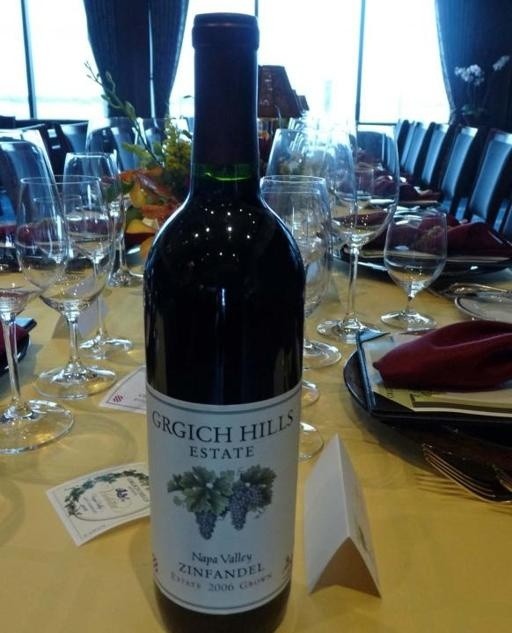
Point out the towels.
[358,176,512,394]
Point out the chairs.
[0,113,167,230]
[392,119,511,231]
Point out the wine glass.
[0,137,75,456]
[60,147,132,357]
[373,206,449,334]
[16,172,118,397]
[83,115,149,286]
[258,114,404,469]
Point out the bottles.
[142,9,306,629]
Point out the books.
[356,331,509,422]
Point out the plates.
[454,291,512,327]
